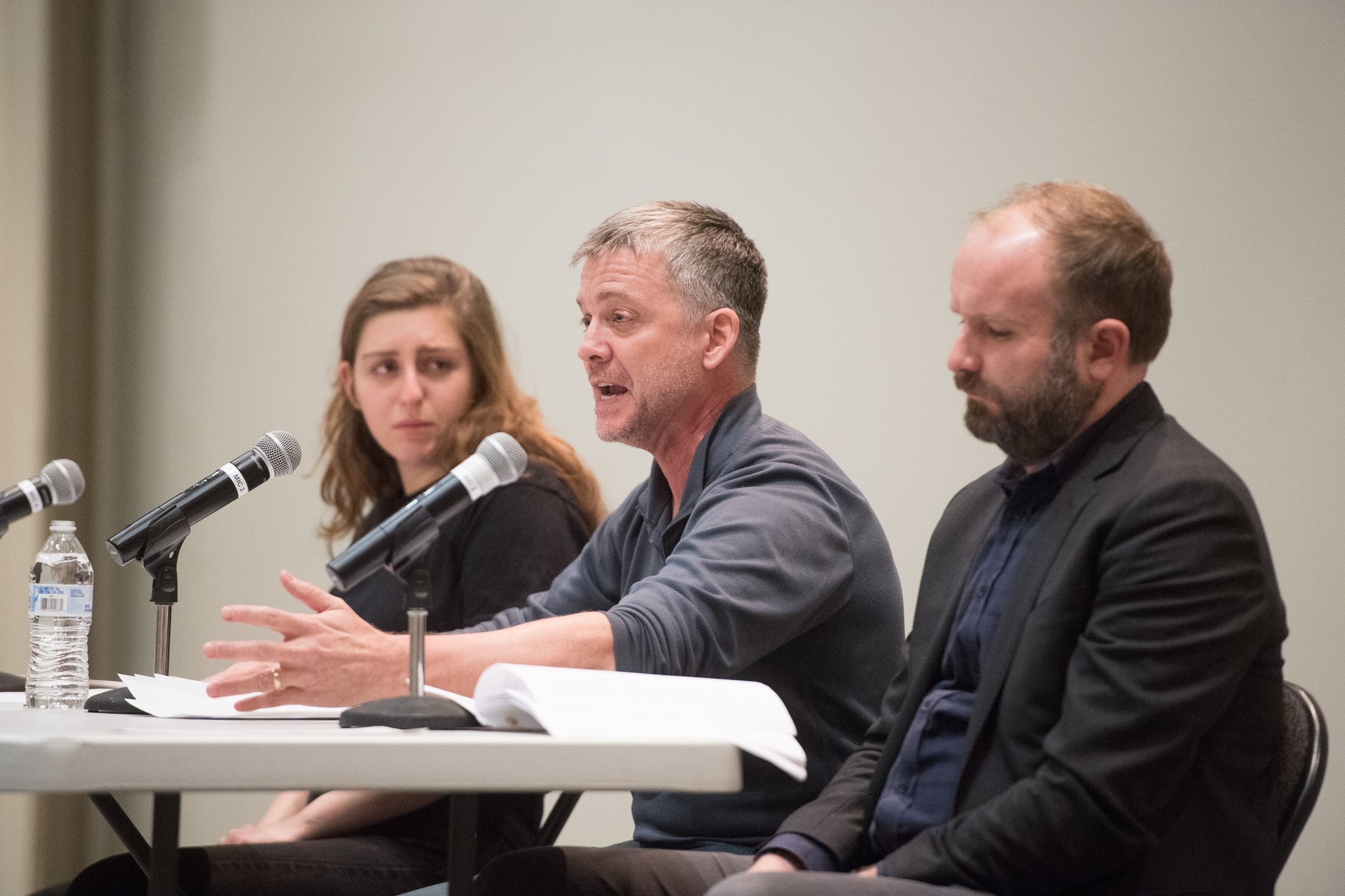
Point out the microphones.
[0,459,85,539]
[326,432,529,591]
[106,431,302,566]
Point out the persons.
[470,182,1289,896]
[28,257,605,896]
[203,201,903,896]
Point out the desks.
[0,680,741,896]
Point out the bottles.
[24,520,94,711]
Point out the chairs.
[1251,677,1328,886]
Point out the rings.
[271,668,282,691]
